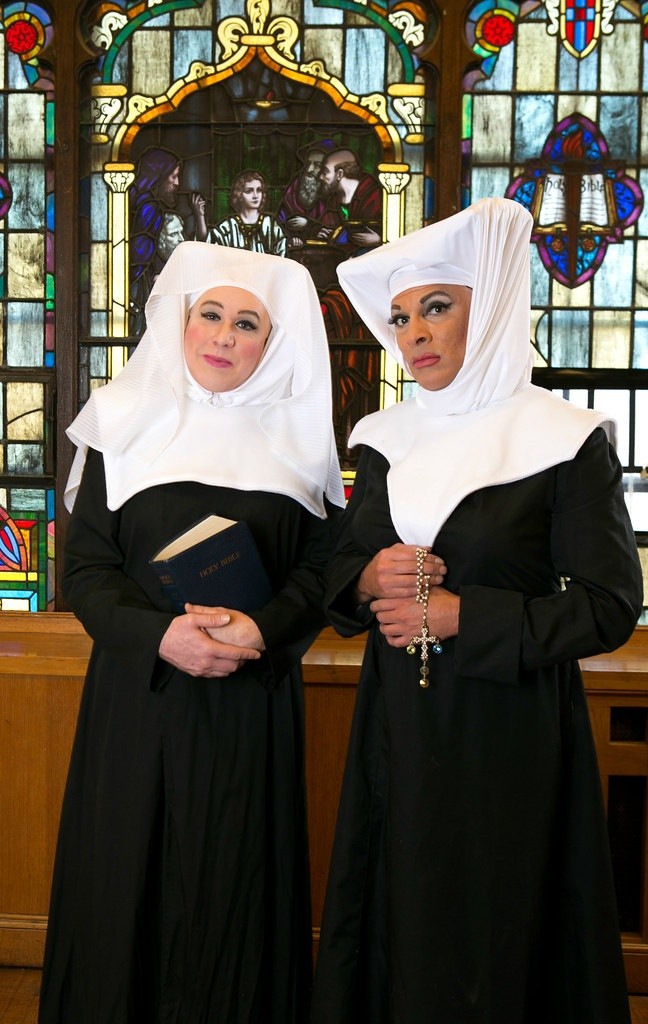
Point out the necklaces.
[406,547,442,690]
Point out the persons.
[36,240,351,1024]
[323,197,643,1024]
